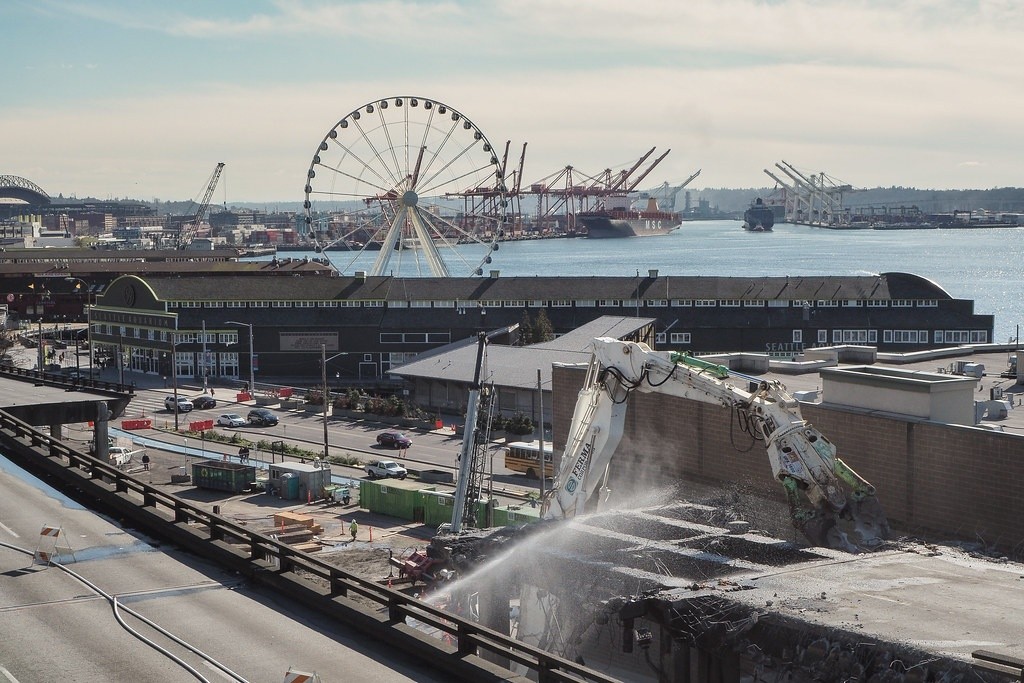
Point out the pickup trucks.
[365,460,407,481]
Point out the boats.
[742,197,775,233]
[580,210,684,238]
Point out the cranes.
[479,137,701,236]
[762,160,853,227]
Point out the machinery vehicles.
[515,334,892,555]
[385,331,500,587]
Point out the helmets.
[352,519,356,524]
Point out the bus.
[504,442,553,480]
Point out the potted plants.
[255,384,330,413]
[332,386,437,429]
[479,410,535,444]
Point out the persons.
[48,348,65,364]
[244,383,248,392]
[239,446,249,464]
[211,386,215,397]
[348,519,358,541]
[142,453,150,471]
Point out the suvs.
[246,408,279,426]
[165,395,194,411]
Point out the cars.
[192,396,217,409]
[377,432,412,448]
[217,413,246,427]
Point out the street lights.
[224,320,255,401]
[66,277,93,381]
[321,343,349,457]
[76,323,102,378]
